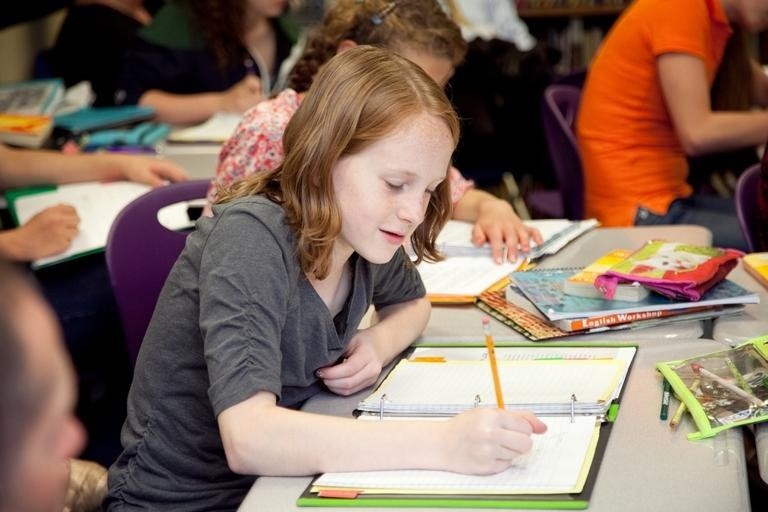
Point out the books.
[472,249,766,341]
[291,338,638,509]
[0,75,182,278]
[398,208,601,308]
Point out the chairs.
[733,164,768,253]
[527,84,586,221]
[105,178,214,367]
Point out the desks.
[164,142,224,183]
[754,423,768,484]
[713,256,768,347]
[356,224,714,338]
[235,337,751,512]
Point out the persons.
[0,144,194,268]
[30,0,768,264]
[95,41,547,510]
[0,255,92,511]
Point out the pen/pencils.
[483,315,504,410]
[505,175,531,222]
[660,363,766,428]
